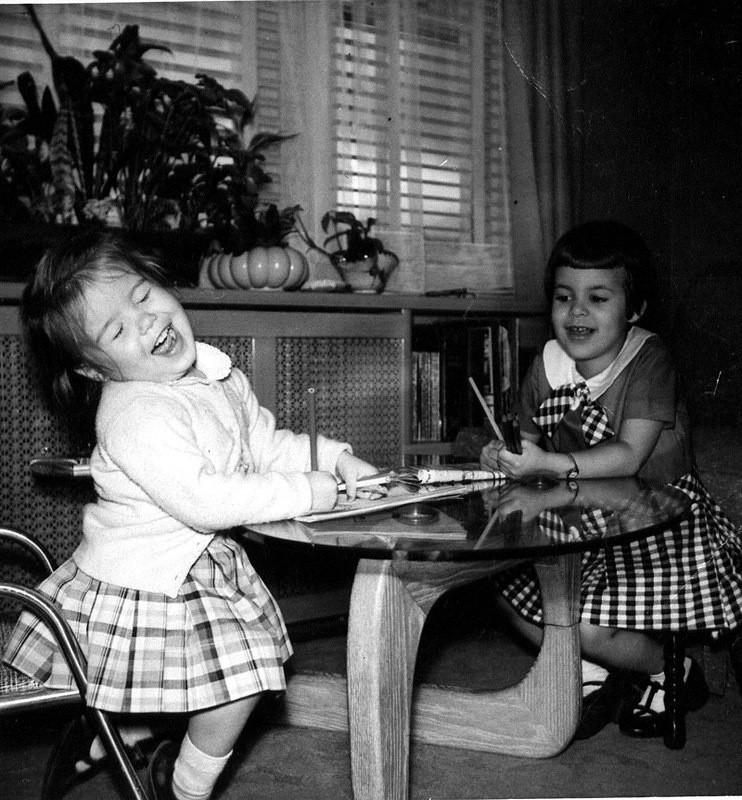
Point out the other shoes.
[41,709,111,800]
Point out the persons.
[481,472,693,543]
[477,218,742,737]
[0,228,386,800]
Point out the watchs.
[566,479,579,507]
[564,452,579,478]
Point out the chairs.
[0,525,157,800]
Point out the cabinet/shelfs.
[0,296,541,624]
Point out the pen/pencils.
[468,376,504,444]
[501,410,525,455]
[473,509,499,551]
[307,387,320,471]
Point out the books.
[290,462,466,523]
[295,507,468,541]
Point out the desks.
[239,484,698,800]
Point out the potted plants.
[299,210,399,294]
[0,4,308,286]
[208,200,305,291]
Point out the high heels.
[574,670,631,739]
[624,658,709,737]
